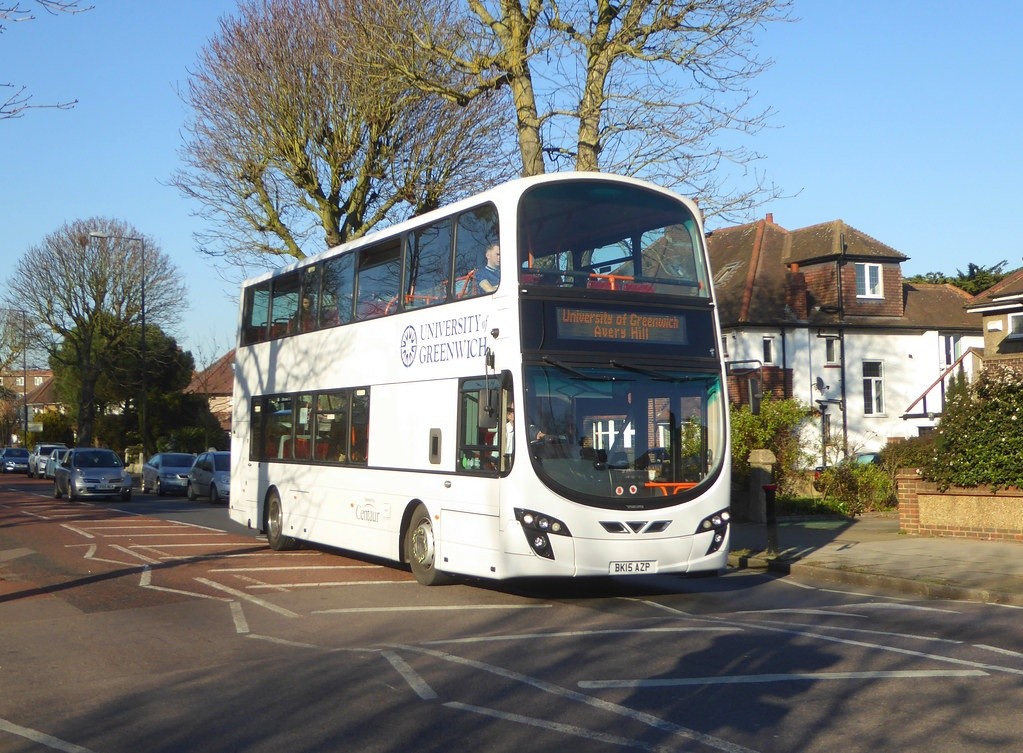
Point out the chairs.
[278,434,330,462]
[258,267,654,344]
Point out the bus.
[228,171,766,586]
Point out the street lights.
[89,231,147,466]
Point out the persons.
[292,292,318,333]
[256,398,367,466]
[476,239,506,296]
[491,398,549,473]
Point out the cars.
[27,445,66,478]
[0,448,30,472]
[54,447,131,501]
[141,453,196,496]
[186,452,230,504]
[813,452,884,491]
[44,449,68,479]
